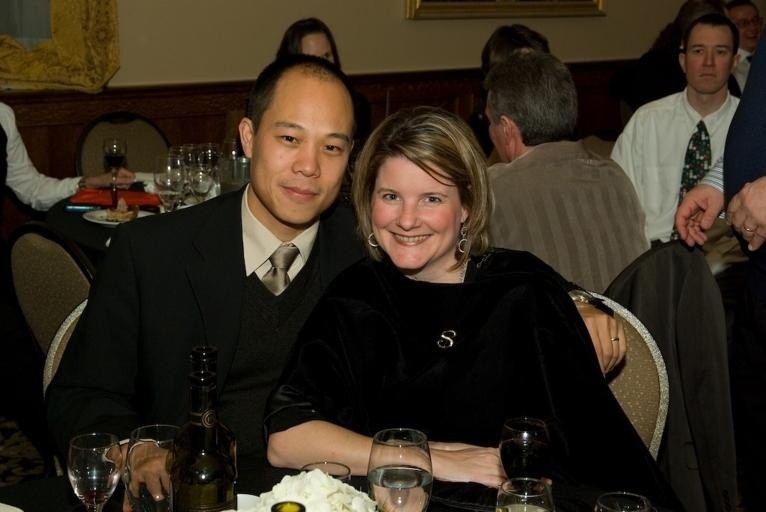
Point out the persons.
[263,104,688,512]
[607,0,766,308]
[466,24,650,295]
[42,51,376,511]
[0,101,135,479]
[236,16,372,158]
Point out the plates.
[80,209,156,229]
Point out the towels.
[70,187,162,206]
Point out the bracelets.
[78,176,86,190]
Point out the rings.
[610,337,620,341]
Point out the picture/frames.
[405,0,608,20]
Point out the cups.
[225,138,239,182]
[302,461,351,483]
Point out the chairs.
[74,111,171,177]
[2,220,100,371]
[42,297,89,400]
[587,290,670,463]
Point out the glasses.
[735,17,760,29]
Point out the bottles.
[272,501,305,512]
[173,345,239,512]
[239,156,249,187]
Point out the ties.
[262,240,300,296]
[671,120,711,241]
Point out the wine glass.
[497,418,552,499]
[594,491,652,512]
[123,424,180,512]
[493,478,568,512]
[153,143,217,213]
[101,137,127,209]
[368,428,434,512]
[67,432,123,512]
